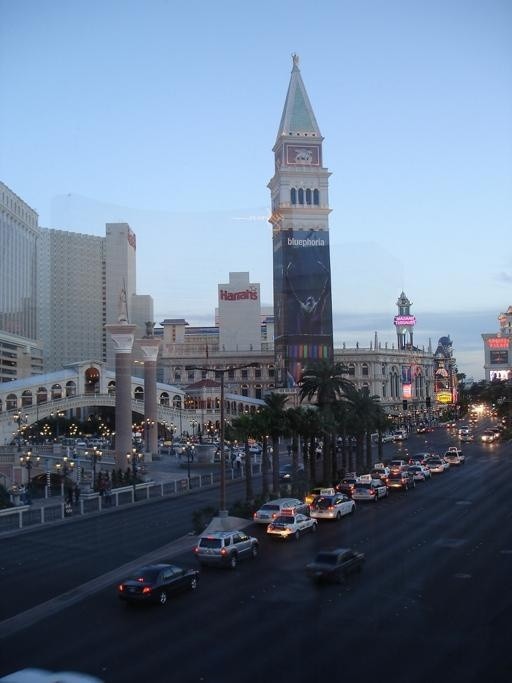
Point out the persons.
[287,260,330,334]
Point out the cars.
[415,404,509,446]
[334,427,407,450]
[0,666,98,682]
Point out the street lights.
[185,361,262,519]
[12,410,245,517]
[387,405,412,425]
